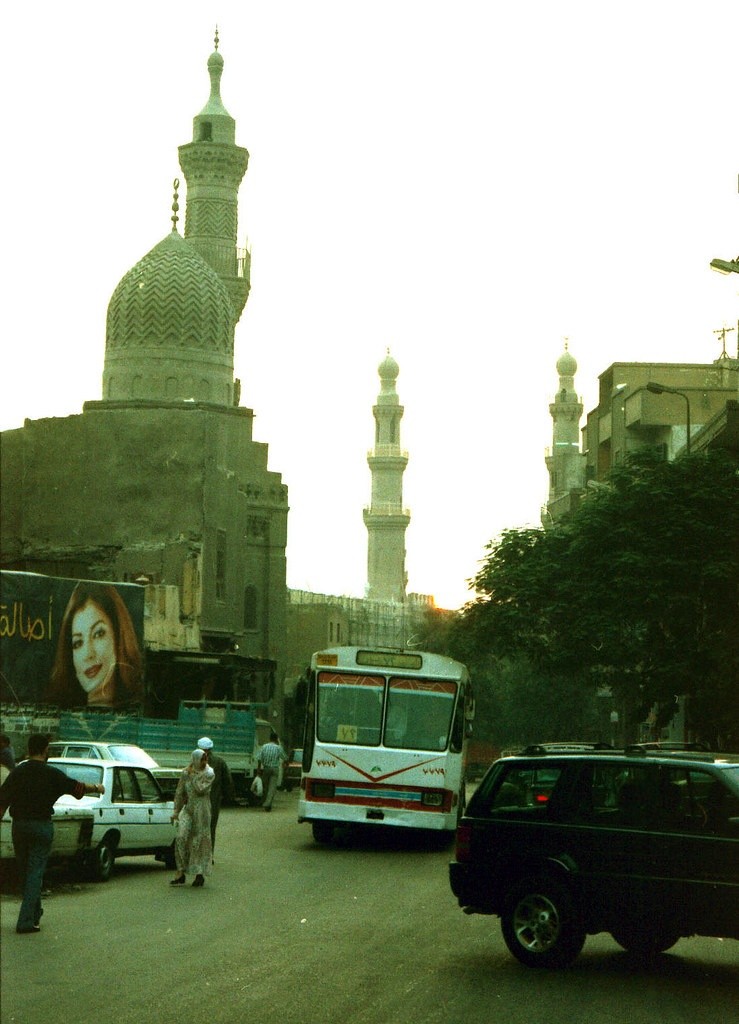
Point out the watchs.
[95,784,99,794]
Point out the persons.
[170,749,214,888]
[256,732,289,812]
[0,733,105,934]
[48,581,144,716]
[197,737,234,864]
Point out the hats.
[197,736,214,752]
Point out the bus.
[297,644,477,845]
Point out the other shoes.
[34,908,45,924]
[169,875,186,886]
[191,875,205,887]
[17,924,41,933]
[266,806,270,812]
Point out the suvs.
[448,740,739,973]
[43,740,185,804]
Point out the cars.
[1,755,179,883]
[283,748,304,785]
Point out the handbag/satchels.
[249,776,263,796]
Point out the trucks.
[0,692,284,809]
